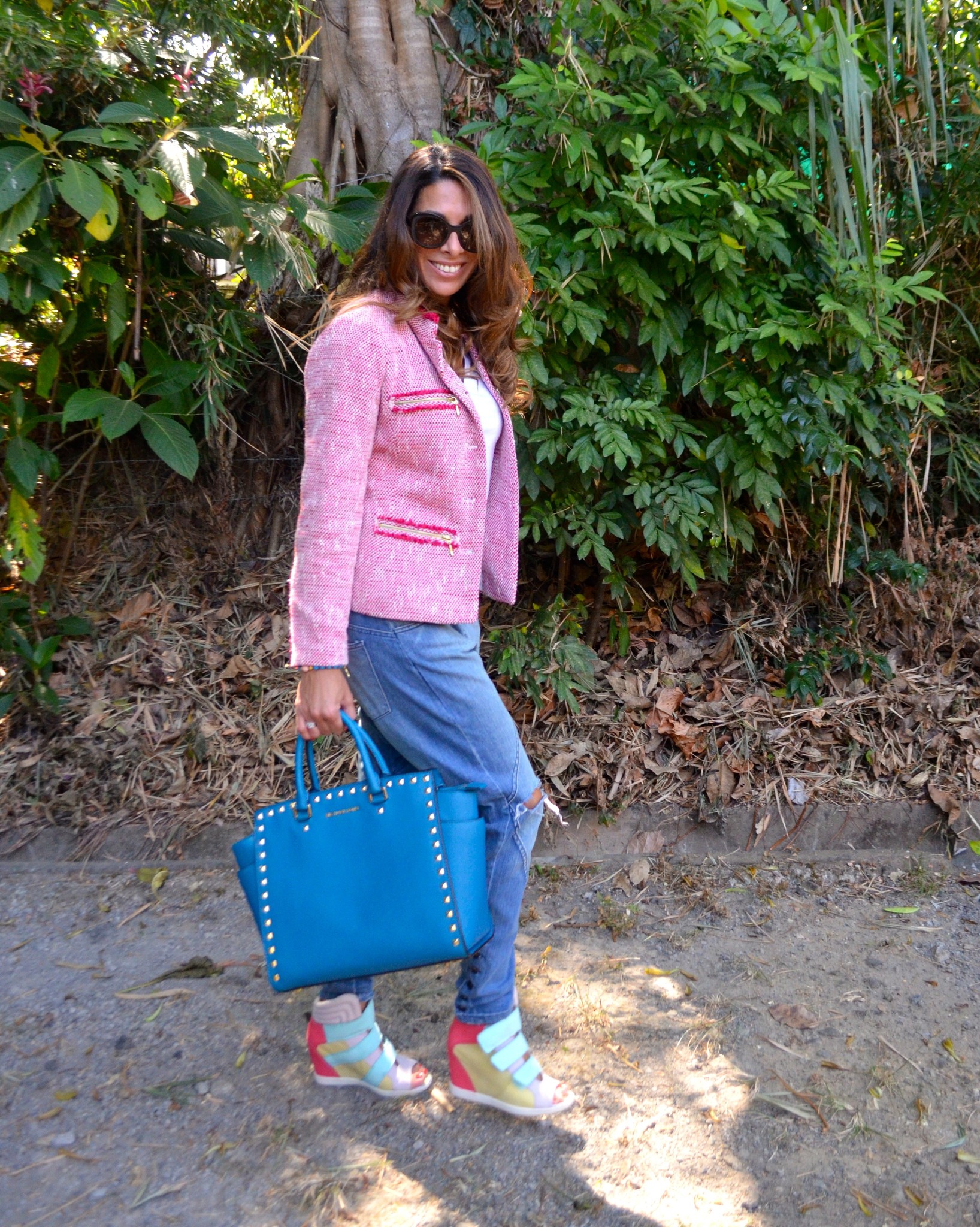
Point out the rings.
[306,722,316,728]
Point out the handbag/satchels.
[231,710,494,990]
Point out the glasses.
[405,211,477,253]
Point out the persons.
[288,140,577,1117]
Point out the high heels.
[303,997,433,1096]
[447,1014,574,1117]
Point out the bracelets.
[295,665,350,672]
